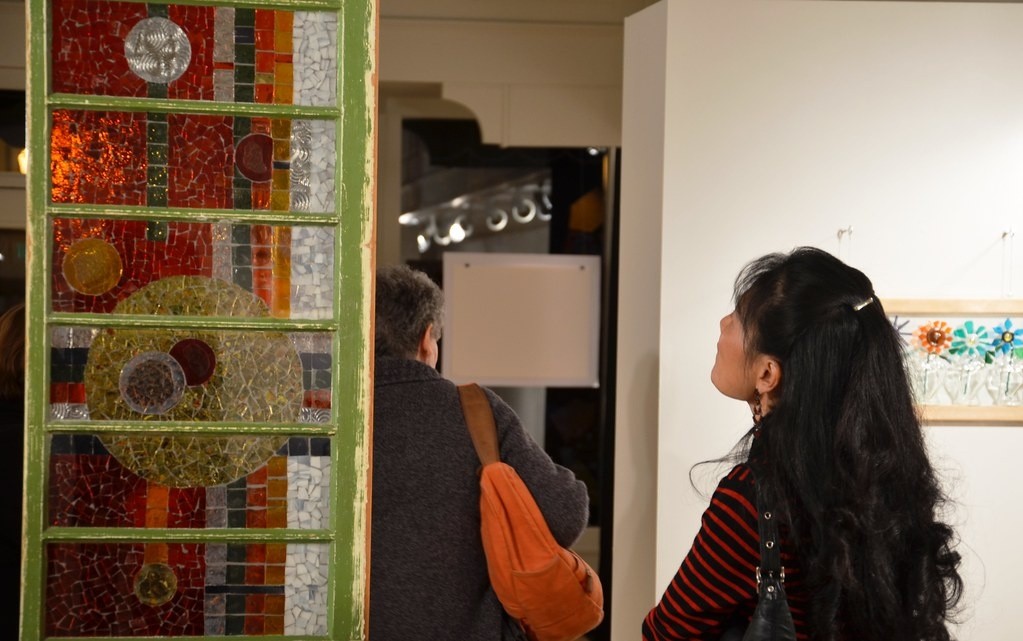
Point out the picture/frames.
[879,301,1023,423]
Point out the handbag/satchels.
[718,461,798,640]
[458,382,604,641]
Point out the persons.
[639,246,962,641]
[369,260,590,641]
